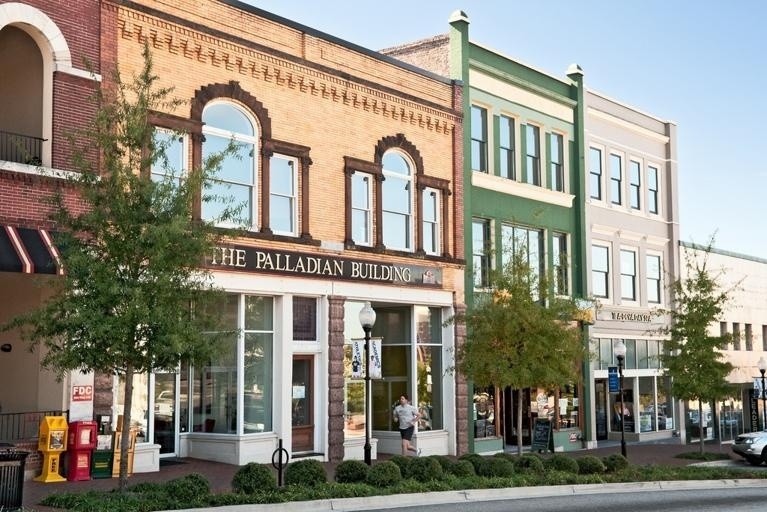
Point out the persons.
[392,394,422,459]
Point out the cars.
[154,389,265,445]
[642,405,741,436]
[731,430,767,465]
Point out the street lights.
[359,301,377,468]
[757,357,767,432]
[614,338,628,460]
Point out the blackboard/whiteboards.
[532,418,551,449]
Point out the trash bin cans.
[0,450,32,512]
